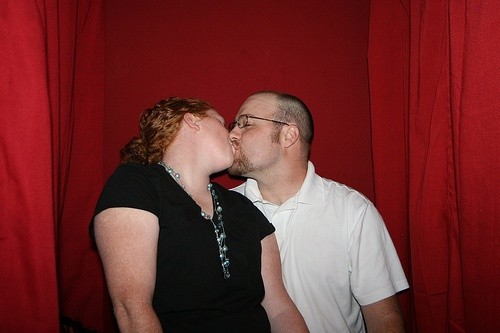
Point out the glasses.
[227,114,290,132]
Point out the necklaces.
[157,159,231,280]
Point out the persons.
[88,96,309,333]
[226,91,411,333]
